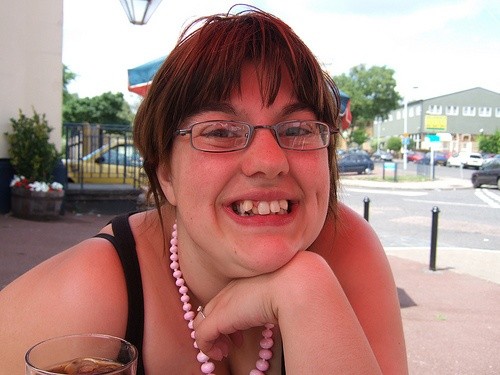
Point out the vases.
[10,188,64,221]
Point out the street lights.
[403,87,418,169]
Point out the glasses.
[172,120,341,154]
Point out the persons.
[0,4,408,375]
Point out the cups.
[25,332,138,375]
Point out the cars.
[337,148,374,175]
[372,151,392,162]
[471,154,500,190]
[406,152,448,166]
[447,152,484,170]
[60,140,150,186]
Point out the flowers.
[4,106,65,193]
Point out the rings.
[197,305,205,318]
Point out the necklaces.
[170,218,275,375]
